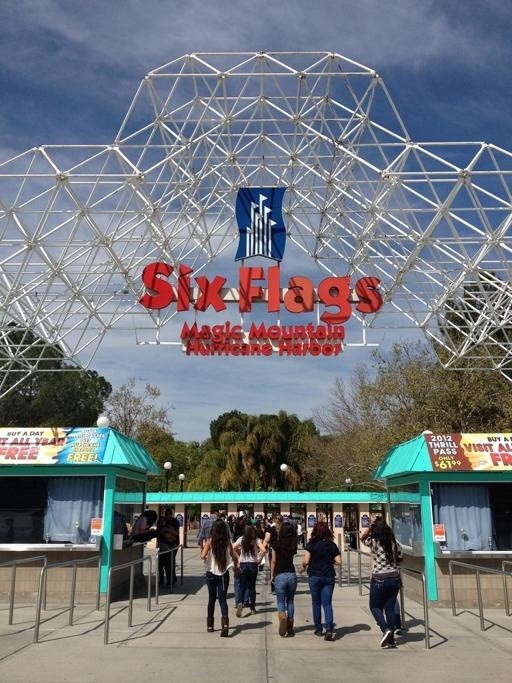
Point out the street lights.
[280,463,288,491]
[345,477,352,491]
[164,461,185,492]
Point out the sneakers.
[379,622,405,649]
[314,628,323,636]
[236,601,244,618]
[250,609,257,615]
[323,630,333,641]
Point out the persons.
[298,521,343,640]
[200,518,234,636]
[384,596,402,635]
[360,516,403,646]
[130,506,308,617]
[269,521,299,636]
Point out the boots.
[277,611,288,637]
[286,618,296,637]
[206,616,216,633]
[219,616,231,638]
